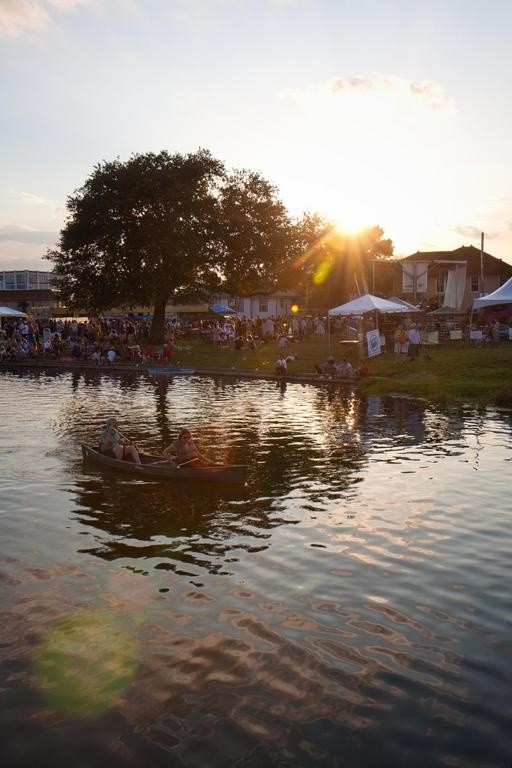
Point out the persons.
[264,313,369,379]
[392,321,421,365]
[164,315,264,358]
[163,430,213,467]
[0,316,155,362]
[99,418,142,464]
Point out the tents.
[328,297,409,356]
[386,295,424,320]
[1,306,27,318]
[468,279,512,338]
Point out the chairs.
[129,343,173,363]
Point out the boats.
[76,439,251,482]
[146,367,199,375]
